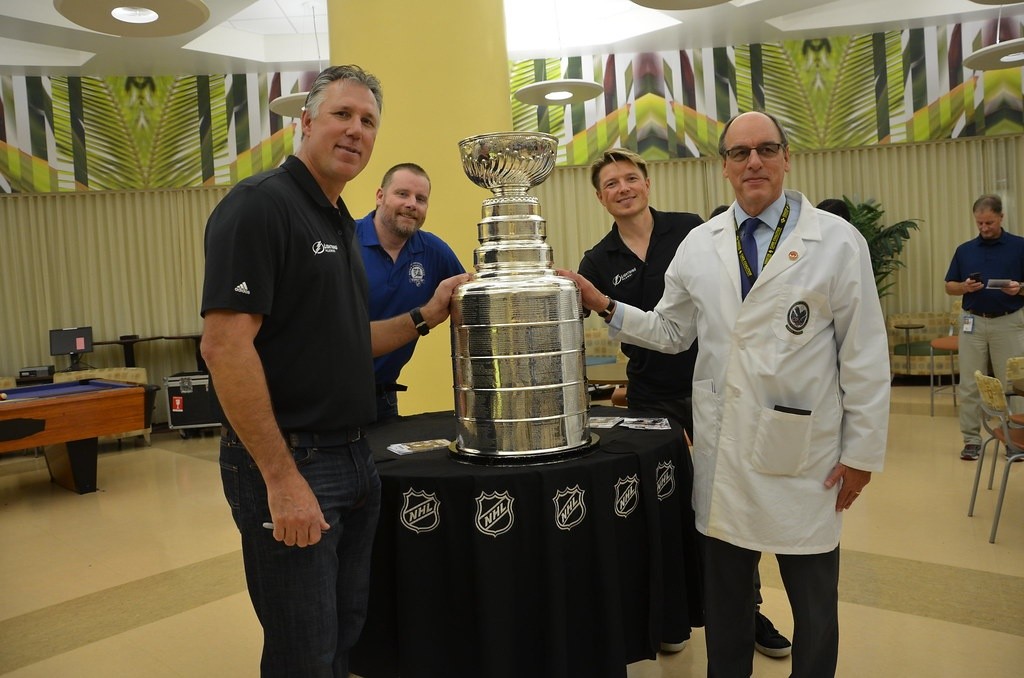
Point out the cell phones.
[970,273,982,283]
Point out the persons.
[553,109,890,678]
[945,194,1024,461]
[197,64,475,678]
[351,164,467,425]
[576,148,794,658]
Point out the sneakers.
[660,631,690,653]
[960,444,981,460]
[1005,445,1024,461]
[752,604,792,657]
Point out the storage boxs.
[165,371,224,428]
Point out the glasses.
[724,144,787,162]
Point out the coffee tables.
[895,323,925,384]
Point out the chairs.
[968,369,1024,543]
[930,295,965,417]
[988,355,1024,491]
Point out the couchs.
[886,310,960,388]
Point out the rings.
[856,491,860,495]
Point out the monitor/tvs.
[49,327,94,371]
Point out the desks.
[360,401,711,678]
[0,378,159,494]
[53,366,154,450]
[92,332,164,375]
[163,333,206,375]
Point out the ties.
[740,219,762,302]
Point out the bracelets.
[409,306,430,336]
[598,295,614,318]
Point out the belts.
[226,428,367,448]
[968,308,1020,319]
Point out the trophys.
[451,130,600,464]
